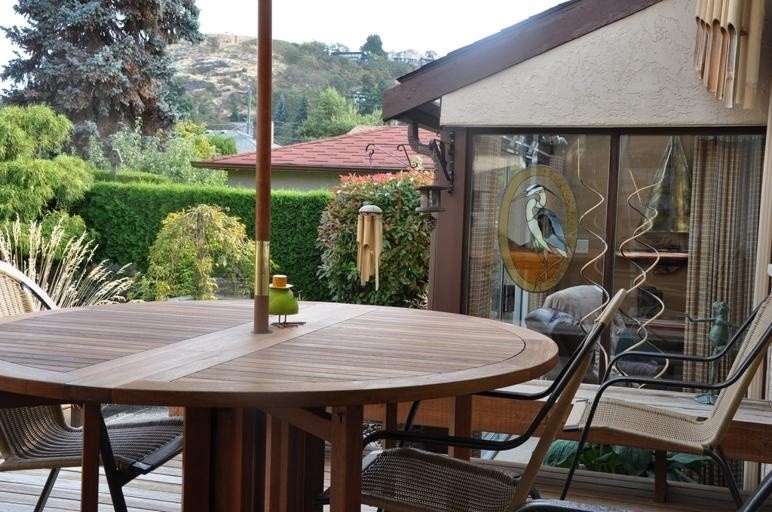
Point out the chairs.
[565,283,772,505]
[361,286,627,508]
[0,261,187,508]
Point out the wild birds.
[511,184,573,291]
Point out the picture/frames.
[498,162,579,292]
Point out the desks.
[0,297,559,506]
